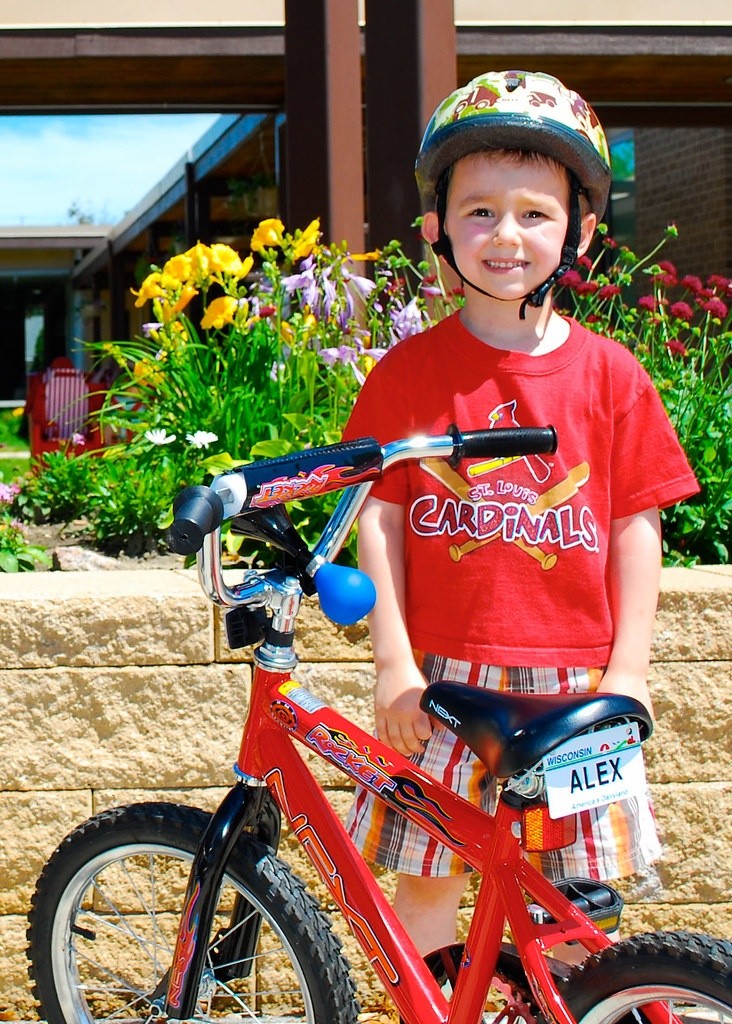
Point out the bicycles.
[26,425,732,1024]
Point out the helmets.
[414,70,612,225]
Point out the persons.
[341,70,701,1003]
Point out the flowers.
[126,216,732,562]
